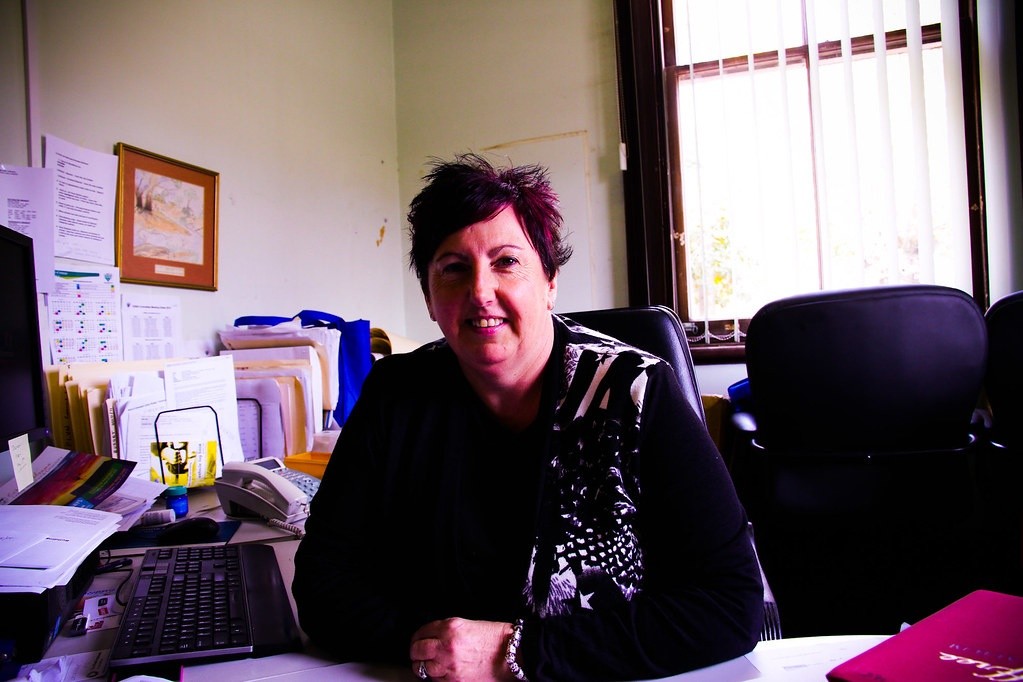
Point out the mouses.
[158,517,221,548]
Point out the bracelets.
[506,619,528,682]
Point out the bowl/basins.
[284,451,332,480]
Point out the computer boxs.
[0,542,100,666]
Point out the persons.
[291,156,764,682]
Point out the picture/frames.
[113,141,221,292]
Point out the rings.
[418,661,428,678]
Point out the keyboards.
[106,544,305,677]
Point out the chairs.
[556,286,1022,637]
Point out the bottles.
[141,509,175,523]
[166,487,188,517]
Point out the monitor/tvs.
[0,224,52,451]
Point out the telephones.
[213,456,323,526]
